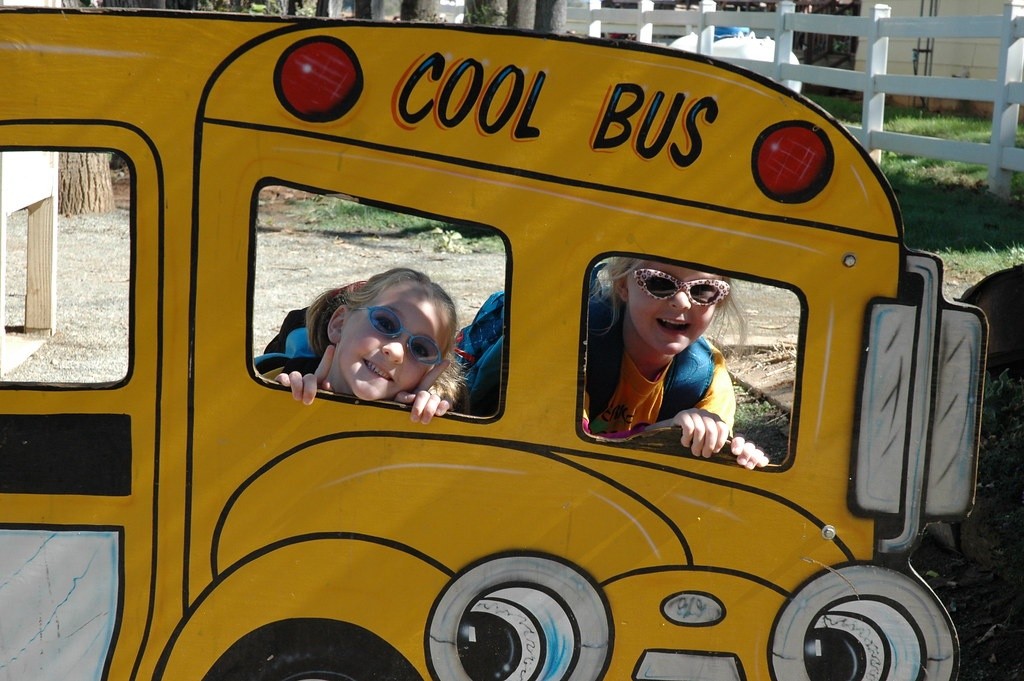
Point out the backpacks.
[453,263,713,423]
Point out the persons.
[454,256,770,471]
[252,268,471,424]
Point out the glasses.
[626,268,731,306]
[351,305,442,366]
